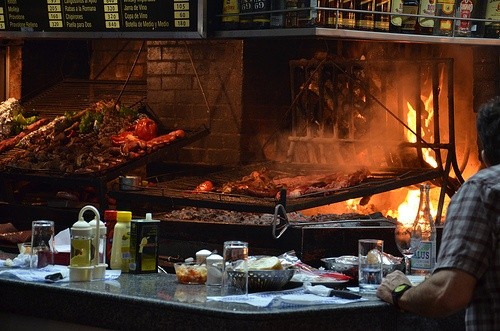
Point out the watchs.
[391,283,413,308]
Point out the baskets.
[230,269,293,293]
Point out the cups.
[196,249,223,285]
[221,241,248,296]
[358,239,384,288]
[30,221,54,276]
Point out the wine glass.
[395,223,422,275]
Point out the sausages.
[235,185,279,198]
[78,129,184,173]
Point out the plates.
[283,280,349,289]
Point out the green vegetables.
[61,105,137,135]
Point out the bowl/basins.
[223,269,294,293]
[320,257,397,285]
[174,262,207,285]
[17,242,31,254]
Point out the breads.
[251,256,282,270]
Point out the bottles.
[68,214,108,282]
[222,0,500,41]
[410,184,436,283]
[104,210,132,273]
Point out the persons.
[376,97,500,331]
